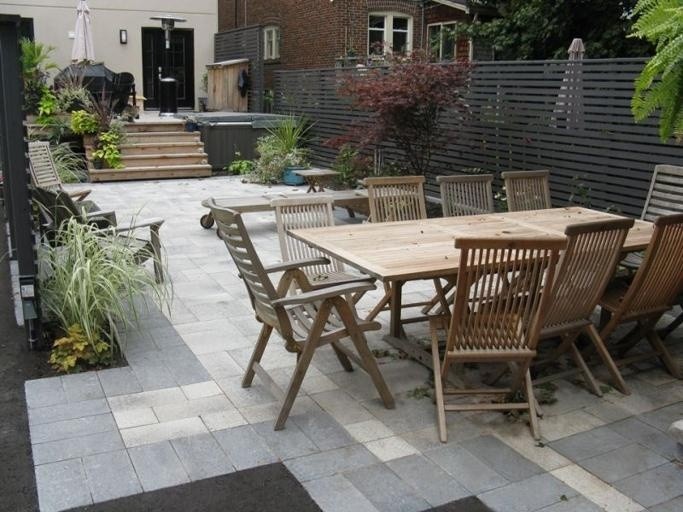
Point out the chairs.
[28,143,165,284]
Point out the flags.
[70,0,96,67]
[549,37,584,134]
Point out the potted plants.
[262,105,320,185]
